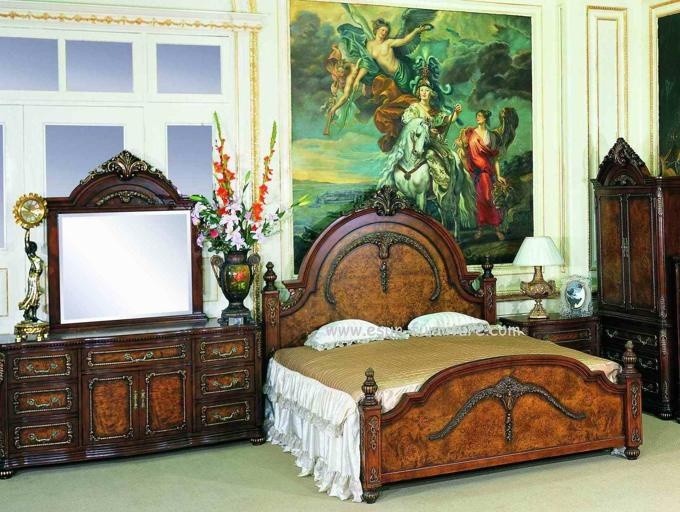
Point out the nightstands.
[500,312,601,357]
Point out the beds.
[261,184,643,505]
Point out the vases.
[210,248,261,327]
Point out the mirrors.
[560,275,591,319]
[39,149,210,335]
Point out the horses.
[375,115,479,242]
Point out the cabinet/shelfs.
[596,310,680,420]
[590,137,679,320]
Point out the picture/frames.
[277,0,567,302]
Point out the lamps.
[511,235,565,319]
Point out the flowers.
[192,112,313,251]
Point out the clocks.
[12,193,49,342]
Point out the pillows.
[302,319,408,351]
[407,311,522,337]
